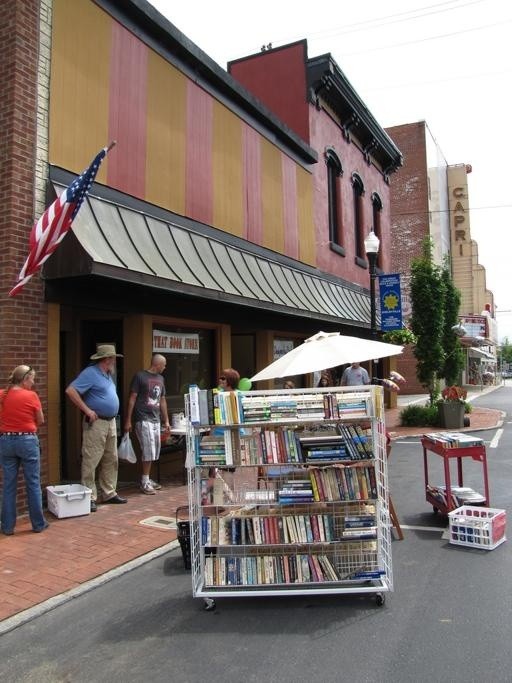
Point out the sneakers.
[139,480,156,495]
[136,479,161,490]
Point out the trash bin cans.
[440,402,465,430]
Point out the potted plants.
[435,382,472,430]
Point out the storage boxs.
[46,483,93,520]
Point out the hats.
[90,344,123,360]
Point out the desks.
[157,426,211,486]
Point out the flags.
[7,148,107,297]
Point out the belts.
[98,415,116,421]
[3,432,36,435]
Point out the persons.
[284,381,295,389]
[340,362,370,386]
[124,354,172,494]
[219,369,240,391]
[65,345,128,512]
[317,375,332,387]
[0,364,49,536]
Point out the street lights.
[363,228,382,382]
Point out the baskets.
[448,505,507,550]
[175,506,191,570]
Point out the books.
[426,484,486,508]
[423,431,483,449]
[189,384,386,585]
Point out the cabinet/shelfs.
[181,381,394,610]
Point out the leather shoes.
[91,500,97,512]
[101,495,126,504]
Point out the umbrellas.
[247,331,405,383]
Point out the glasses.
[23,366,32,379]
[219,377,226,380]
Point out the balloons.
[237,378,251,391]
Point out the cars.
[497,368,512,378]
